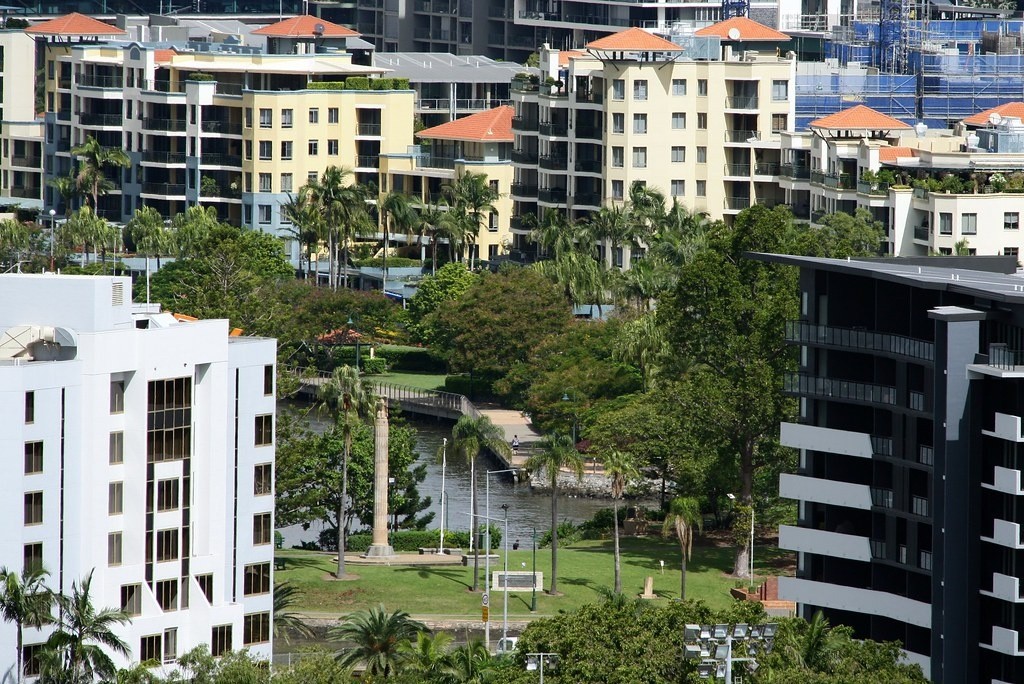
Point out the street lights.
[483,467,526,654]
[49,209,56,273]
[457,511,510,651]
[440,437,447,555]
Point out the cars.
[495,638,522,655]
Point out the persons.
[512,435,519,455]
